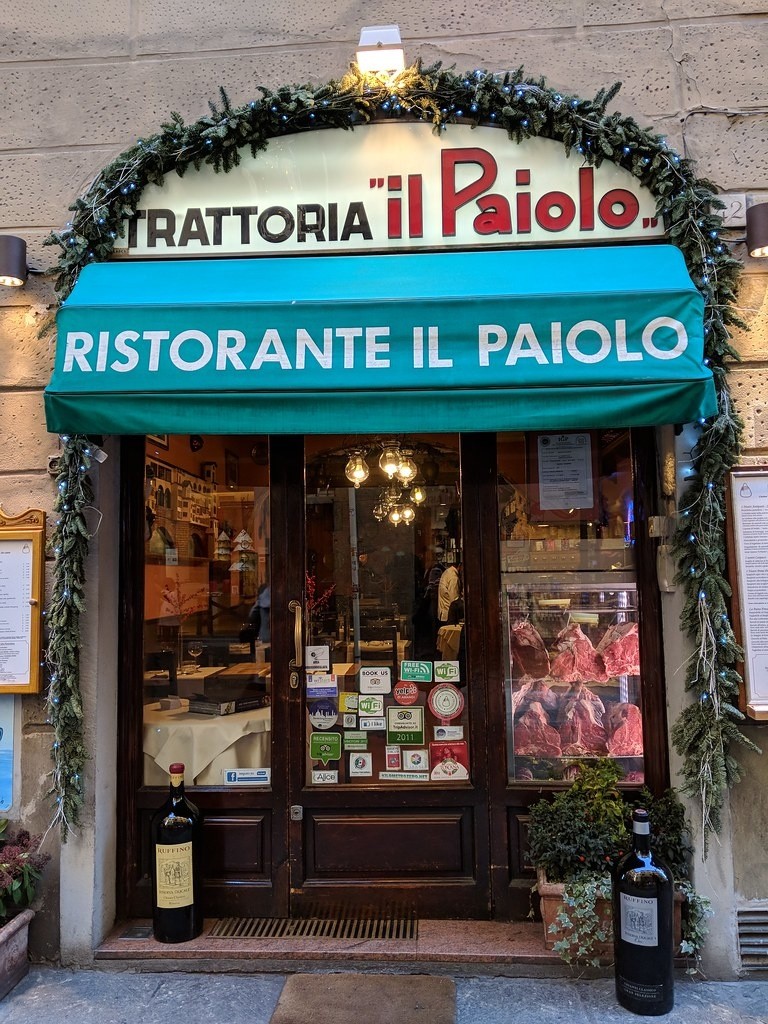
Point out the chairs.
[181,623,257,668]
[360,626,398,687]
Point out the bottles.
[611,809,676,1015]
[153,763,202,943]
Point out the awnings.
[40,242,720,438]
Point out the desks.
[436,624,463,661]
[231,642,271,662]
[142,699,318,785]
[344,640,413,680]
[143,661,361,702]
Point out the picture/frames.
[146,434,169,451]
[225,449,239,492]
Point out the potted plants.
[0,818,52,1001]
[525,754,715,983]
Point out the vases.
[307,622,315,646]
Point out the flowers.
[305,569,337,621]
[163,573,209,625]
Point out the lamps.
[342,433,441,526]
[356,23,406,74]
[0,235,30,288]
[736,202,768,258]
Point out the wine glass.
[188,641,203,673]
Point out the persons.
[447,583,465,625]
[437,561,462,662]
[248,583,271,643]
